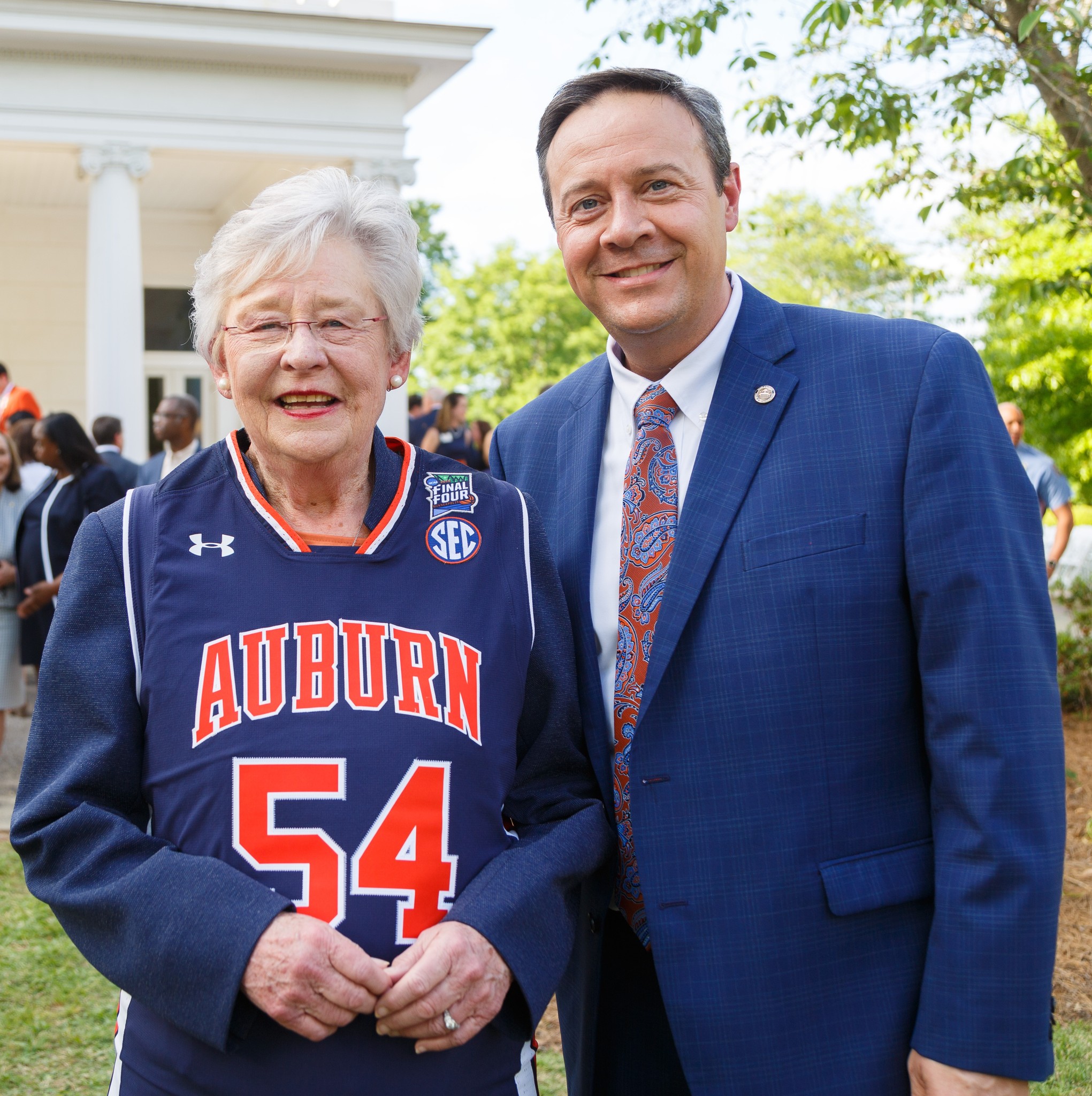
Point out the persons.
[0,363,499,717]
[8,159,617,1096]
[996,401,1075,584]
[484,68,1066,1096]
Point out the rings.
[441,1009,460,1031]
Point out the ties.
[613,383,685,953]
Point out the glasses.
[220,312,387,354]
[154,411,182,419]
